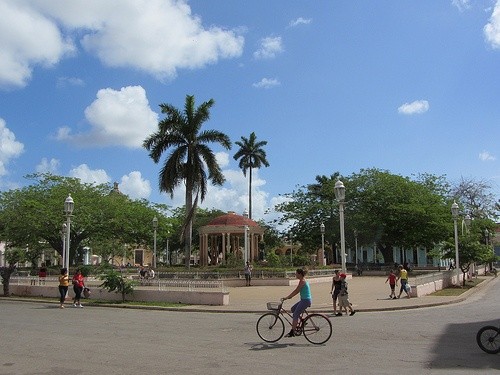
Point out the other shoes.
[350,310,356,316]
[336,313,342,316]
[393,296,396,299]
[61,306,64,309]
[78,304,83,307]
[284,330,295,337]
[389,295,392,298]
[72,303,77,307]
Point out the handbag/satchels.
[83,288,91,298]
[405,283,412,293]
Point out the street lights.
[451,199,461,287]
[62,193,74,275]
[354,228,359,264]
[485,229,489,247]
[290,231,293,267]
[152,214,158,268]
[334,177,347,273]
[243,209,250,266]
[320,222,325,268]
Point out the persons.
[385,270,398,299]
[330,270,348,316]
[438,264,441,272]
[280,268,312,338]
[39,263,46,281]
[396,264,410,298]
[335,274,356,316]
[448,259,454,271]
[72,268,86,308]
[147,266,155,279]
[244,261,253,286]
[58,268,69,309]
[139,267,148,279]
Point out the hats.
[339,274,346,277]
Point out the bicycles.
[256,298,332,345]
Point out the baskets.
[267,302,282,310]
